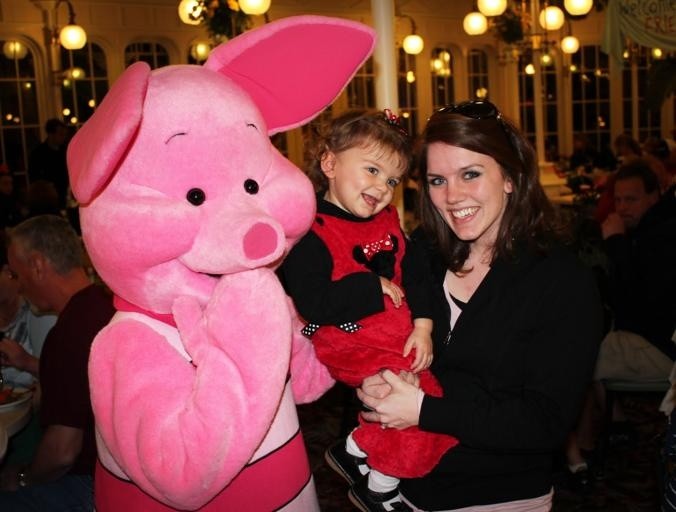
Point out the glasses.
[439,100,498,120]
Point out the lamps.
[179,0,272,65]
[461,1,667,79]
[401,33,452,85]
[6,22,98,127]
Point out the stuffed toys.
[65,14,376,511]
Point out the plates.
[0,386,33,414]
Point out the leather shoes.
[325,439,413,512]
